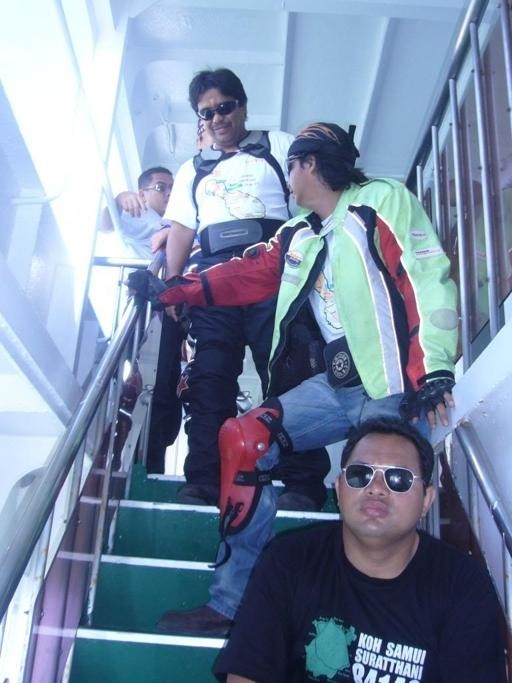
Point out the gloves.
[126,268,166,303]
[416,369,456,414]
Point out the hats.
[287,122,355,164]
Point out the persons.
[120,121,461,639]
[212,416,509,683]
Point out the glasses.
[196,97,239,120]
[340,463,423,494]
[140,182,167,192]
[283,154,306,173]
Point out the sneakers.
[178,483,220,503]
[276,493,320,511]
[155,604,235,639]
